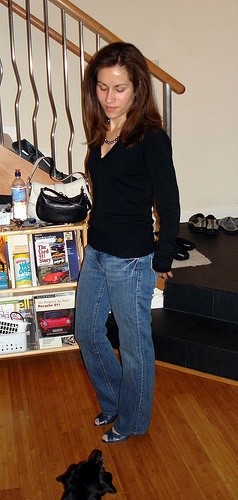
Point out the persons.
[74,42,181,444]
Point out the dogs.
[55,448,117,500]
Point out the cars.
[54,260,62,264]
[57,238,62,242]
[40,311,72,337]
[52,253,62,258]
[52,256,62,259]
[42,267,69,283]
[60,243,63,245]
[51,250,59,254]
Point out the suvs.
[51,244,64,252]
[18,317,36,350]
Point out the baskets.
[0,317,32,354]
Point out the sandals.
[94,412,116,425]
[102,426,129,442]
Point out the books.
[0,235,37,350]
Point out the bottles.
[11,169,28,221]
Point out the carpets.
[171,248,212,269]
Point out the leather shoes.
[174,237,194,260]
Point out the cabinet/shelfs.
[0,219,90,358]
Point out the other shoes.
[189,212,206,232]
[206,214,219,234]
[219,216,238,232]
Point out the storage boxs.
[33,230,80,286]
[0,316,31,355]
[39,334,79,350]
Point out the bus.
[37,236,56,243]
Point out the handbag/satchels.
[36,187,91,224]
[27,156,94,223]
